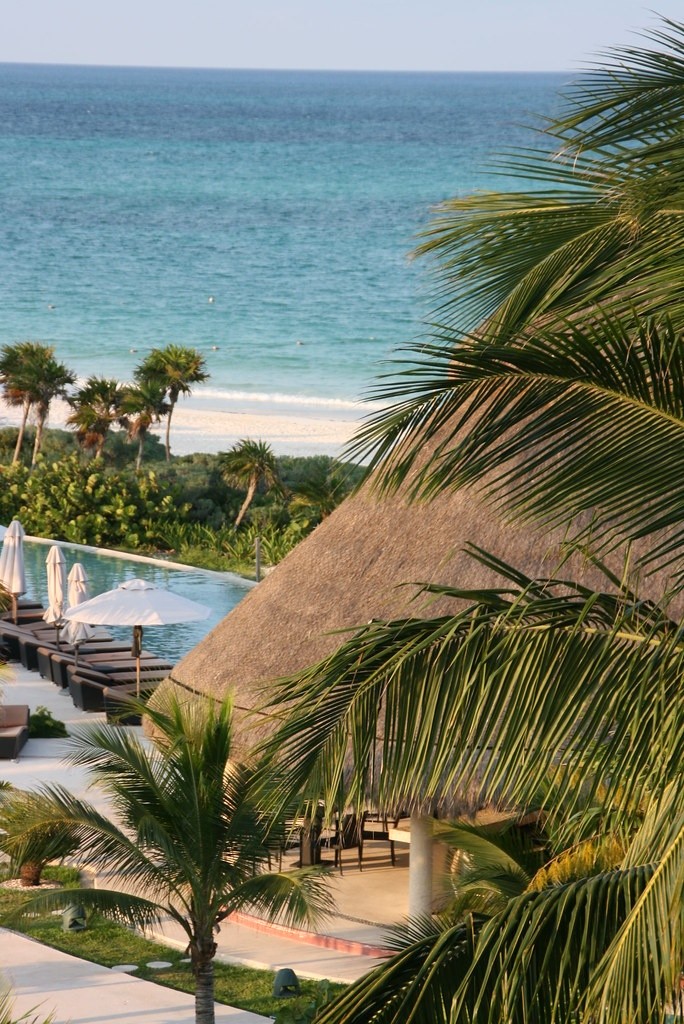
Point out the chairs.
[265,810,409,876]
[0,704,30,760]
[0,598,176,727]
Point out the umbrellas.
[138,252,684,814]
[0,520,27,624]
[63,579,210,698]
[60,563,95,665]
[42,546,68,648]
